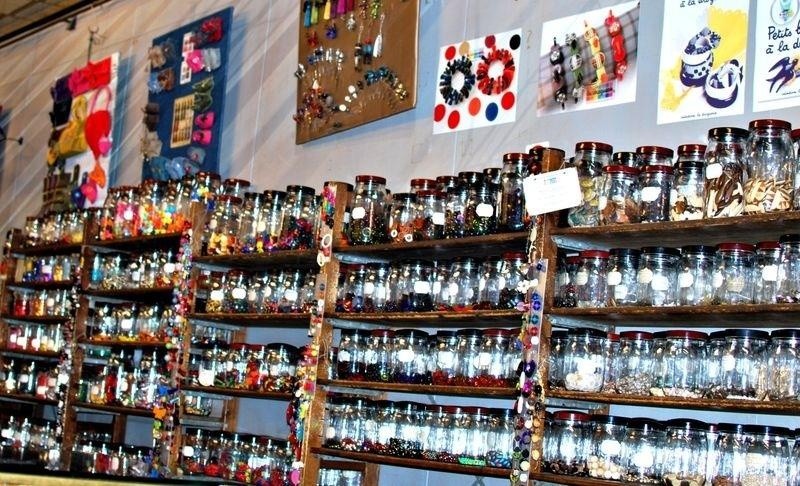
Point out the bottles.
[650,331,666,397]
[613,152,637,168]
[347,145,545,247]
[677,244,716,306]
[336,251,526,314]
[193,268,325,314]
[542,411,552,470]
[791,128,800,210]
[602,333,619,393]
[628,417,664,486]
[721,329,770,401]
[198,177,332,256]
[22,206,88,247]
[637,146,674,166]
[76,350,166,410]
[663,330,708,398]
[574,250,609,308]
[90,301,175,340]
[742,425,790,486]
[0,415,61,471]
[704,127,749,218]
[587,415,628,480]
[5,323,65,355]
[181,424,297,486]
[614,331,653,396]
[664,419,708,486]
[487,407,514,468]
[567,141,613,227]
[767,329,800,401]
[637,165,673,222]
[709,330,727,400]
[549,331,568,390]
[637,246,680,307]
[600,165,638,226]
[92,246,176,289]
[747,119,795,210]
[563,328,607,393]
[8,286,80,317]
[69,433,155,477]
[778,234,800,303]
[719,242,755,306]
[549,410,590,476]
[713,423,743,486]
[22,252,81,283]
[3,359,60,400]
[324,396,425,458]
[605,248,641,306]
[676,145,706,162]
[99,169,222,241]
[188,334,310,394]
[458,406,487,466]
[336,328,522,388]
[790,428,800,486]
[755,242,781,304]
[442,405,462,462]
[421,404,443,461]
[669,161,704,222]
[565,256,581,308]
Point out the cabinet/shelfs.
[1,146,800,486]
